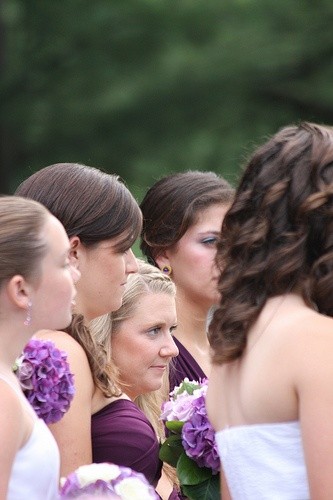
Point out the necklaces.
[175,315,213,361]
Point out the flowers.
[157,376,223,500]
[11,339,76,426]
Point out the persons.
[138,170,239,401]
[1,194,82,499]
[205,120,333,500]
[15,160,162,499]
[62,255,185,500]
